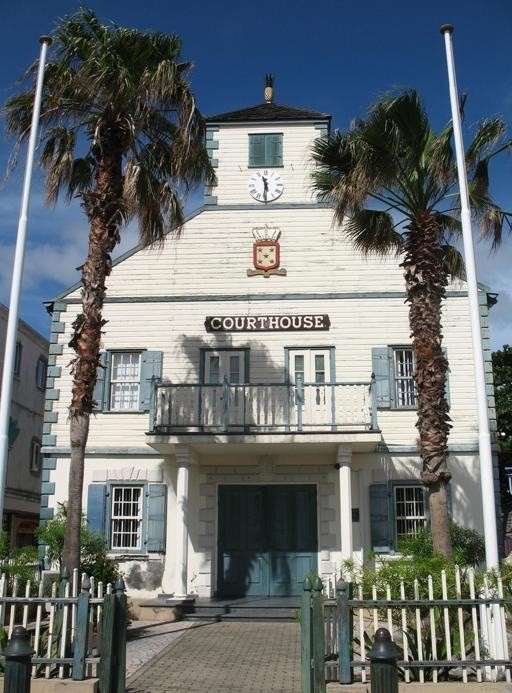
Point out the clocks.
[248,169,284,204]
